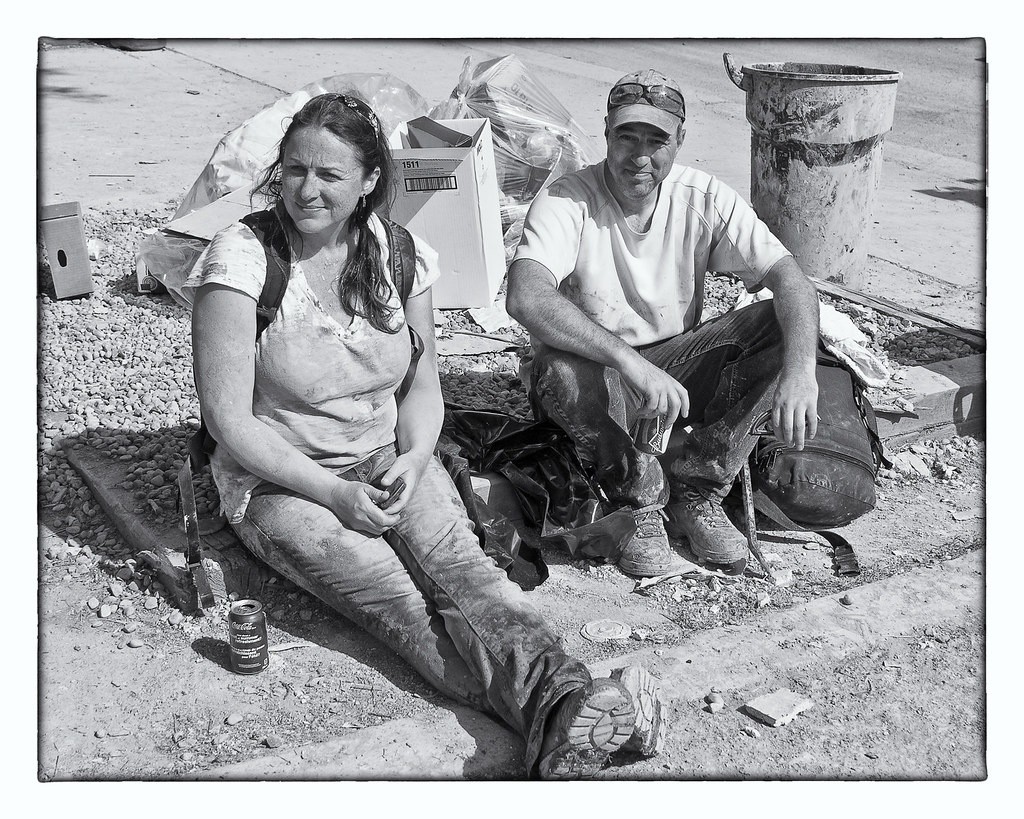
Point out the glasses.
[606,82,686,124]
[748,408,822,435]
[311,92,381,149]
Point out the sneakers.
[664,497,750,564]
[618,508,671,577]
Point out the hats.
[606,68,685,135]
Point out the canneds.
[632,400,673,456]
[228,599,270,675]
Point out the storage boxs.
[466,53,571,146]
[384,115,507,310]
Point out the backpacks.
[748,340,893,529]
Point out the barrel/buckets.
[723,52,903,291]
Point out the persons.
[505,70,819,577]
[182,93,669,777]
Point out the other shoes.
[608,665,667,756]
[538,677,635,781]
